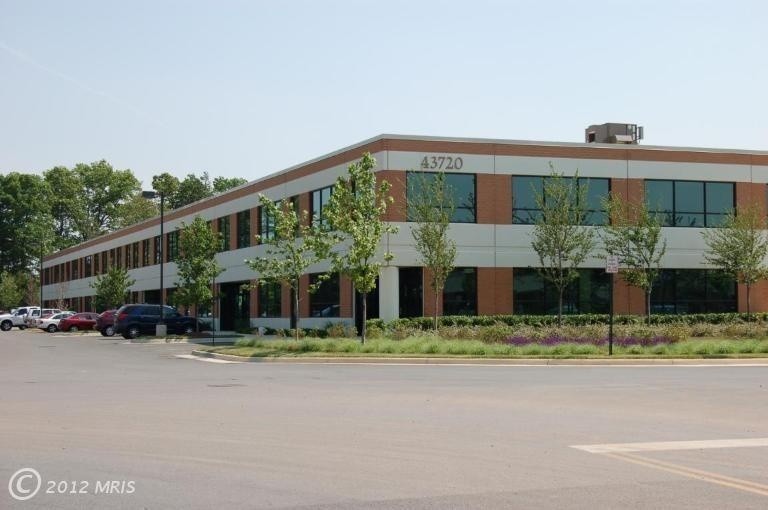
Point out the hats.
[95,310,116,337]
[314,304,339,317]
[59,313,100,332]
[37,313,75,333]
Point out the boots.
[113,303,202,339]
[23,308,63,327]
[0,307,40,331]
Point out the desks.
[140,190,165,325]
